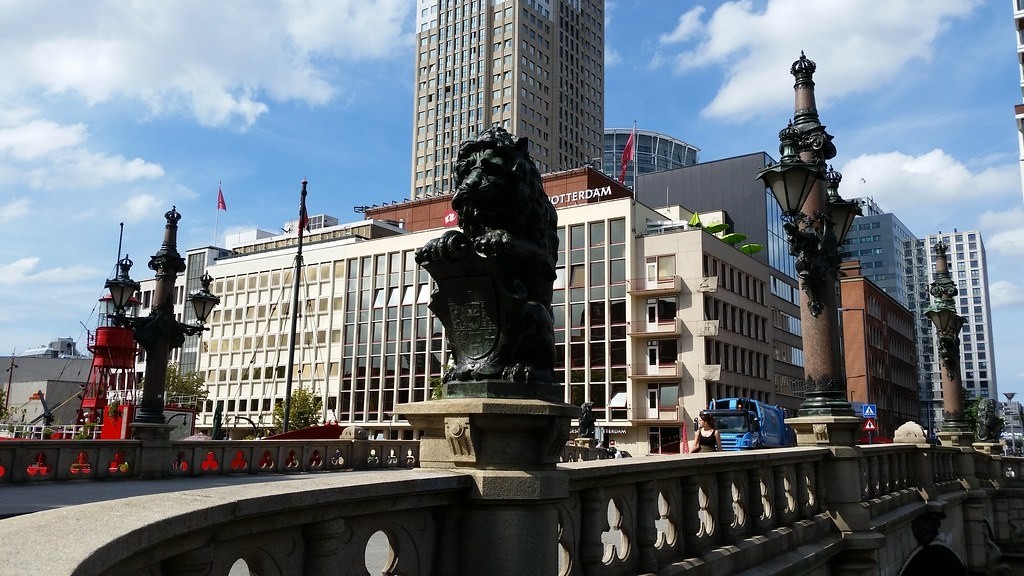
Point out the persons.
[693,413,723,453]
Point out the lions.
[976,398,1005,443]
[414,126,560,397]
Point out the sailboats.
[0,178,349,482]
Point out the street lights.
[1002,392,1018,453]
[922,238,975,446]
[754,47,863,446]
[103,203,222,448]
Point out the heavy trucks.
[693,397,797,453]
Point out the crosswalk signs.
[861,404,876,419]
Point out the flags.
[297,202,311,236]
[619,129,633,185]
[216,188,227,211]
